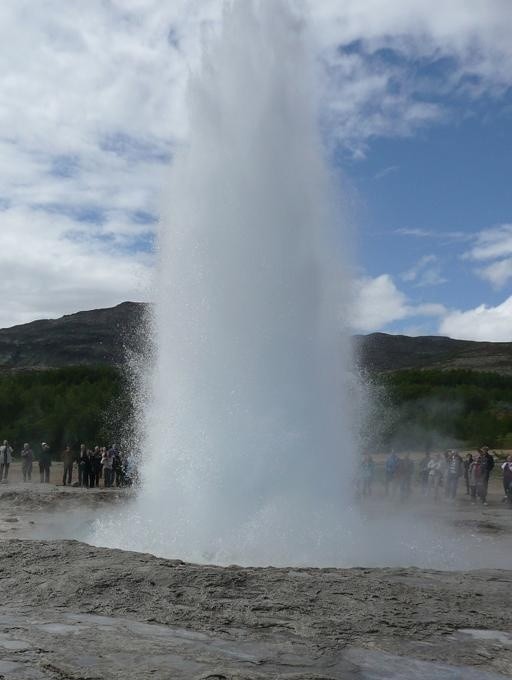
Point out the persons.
[21,444,33,483]
[0,440,13,481]
[38,443,52,483]
[60,442,139,489]
[360,446,510,506]
